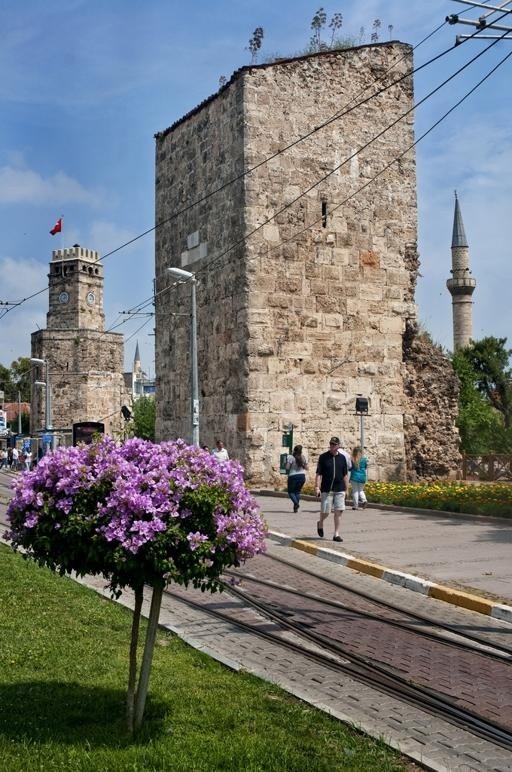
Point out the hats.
[329,437,340,445]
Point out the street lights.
[349,397,372,484]
[166,267,200,452]
[33,379,52,428]
[29,357,49,430]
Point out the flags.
[49,218,61,236]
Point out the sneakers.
[352,501,368,510]
[294,505,299,512]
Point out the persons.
[199,440,230,461]
[0,444,35,471]
[285,437,368,542]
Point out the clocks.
[58,292,69,304]
[87,293,96,306]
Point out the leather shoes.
[317,521,324,537]
[333,536,343,542]
